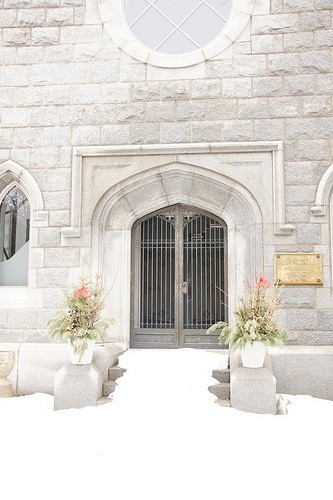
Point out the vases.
[239,340,266,369]
[66,340,94,365]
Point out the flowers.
[205,269,293,348]
[45,265,117,345]
